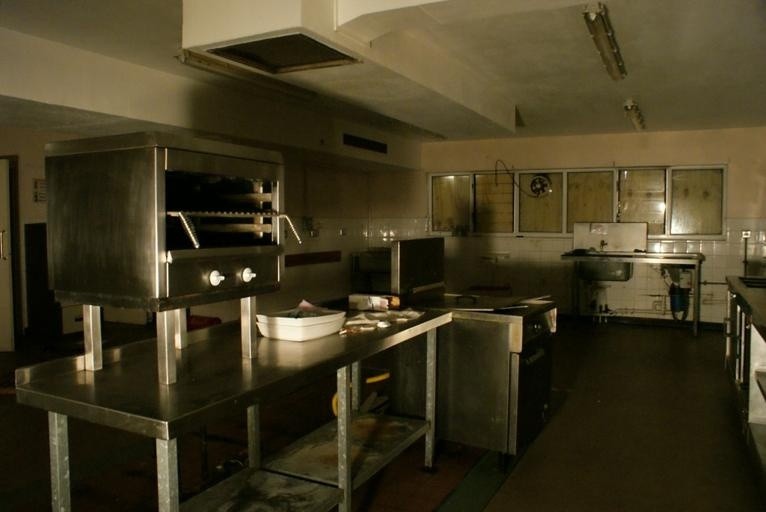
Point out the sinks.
[574,252,634,282]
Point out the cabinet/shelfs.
[389,302,560,472]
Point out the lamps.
[173,48,316,103]
[583,2,626,82]
[623,99,646,133]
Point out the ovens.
[37,135,288,312]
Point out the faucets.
[599,239,608,250]
